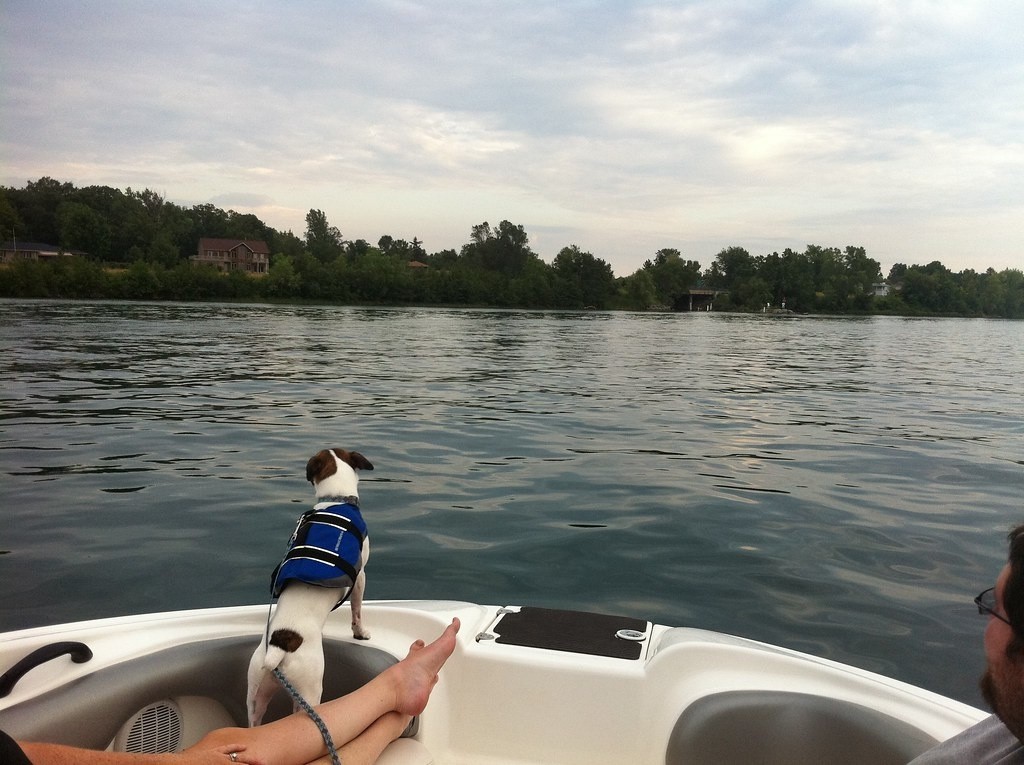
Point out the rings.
[229,753,237,761]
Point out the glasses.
[975,587,1020,632]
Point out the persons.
[906,525,1024,764]
[0,618,461,764]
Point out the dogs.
[246,447,374,729]
